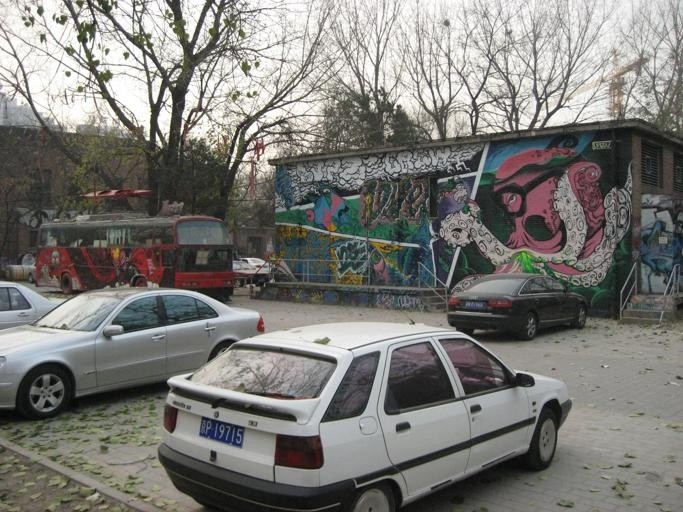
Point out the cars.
[446,272,588,339]
[27,263,36,282]
[233,256,274,287]
[0,281,265,420]
[157,320,573,512]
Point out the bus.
[35,211,236,295]
